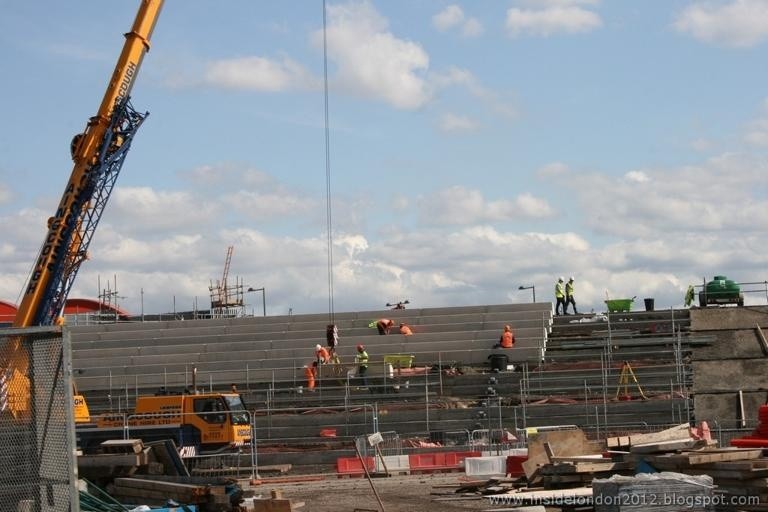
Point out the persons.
[565,275,581,315]
[329,348,343,374]
[315,344,330,365]
[554,276,571,316]
[354,345,370,391]
[377,317,394,335]
[302,365,314,391]
[492,325,516,350]
[229,383,238,395]
[399,322,413,336]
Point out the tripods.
[615,361,645,401]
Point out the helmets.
[504,324,512,333]
[356,344,364,350]
[559,277,564,283]
[315,343,321,352]
[569,277,575,280]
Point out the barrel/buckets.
[490,354,507,372]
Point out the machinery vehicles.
[0,0,254,474]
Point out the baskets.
[383,354,416,369]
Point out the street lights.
[248,288,265,317]
[519,286,536,301]
